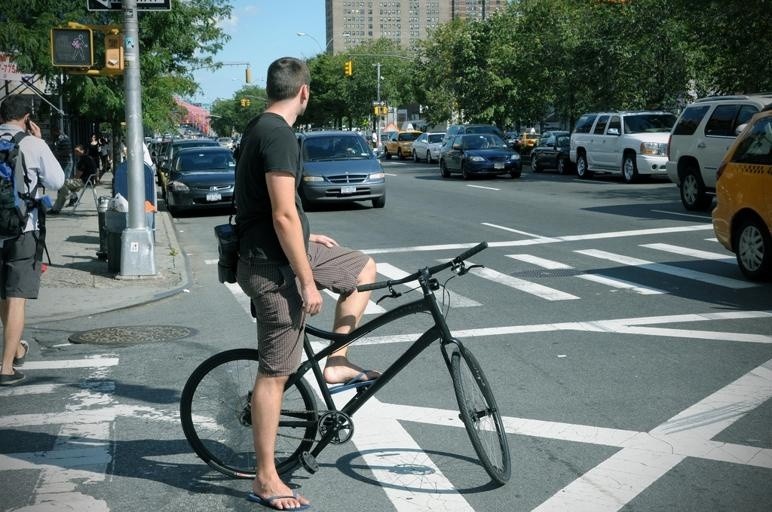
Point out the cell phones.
[24,118,32,129]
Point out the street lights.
[295,28,353,55]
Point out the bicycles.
[178,240,519,491]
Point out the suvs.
[666,95,772,209]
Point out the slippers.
[326,371,382,395]
[249,491,312,511]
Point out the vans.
[569,111,689,177]
[294,130,390,211]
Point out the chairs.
[68,174,99,216]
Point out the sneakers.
[0,368,25,385]
[13,340,29,366]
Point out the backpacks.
[0,132,40,235]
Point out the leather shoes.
[47,196,78,214]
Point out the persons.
[47,129,112,217]
[235,57,383,510]
[372,130,377,148]
[0,95,66,385]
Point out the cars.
[710,109,769,279]
[410,132,455,163]
[528,133,584,173]
[438,134,523,180]
[158,147,245,214]
[145,136,233,165]
[385,130,426,162]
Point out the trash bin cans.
[103,209,154,275]
[98,211,107,260]
[112,159,155,206]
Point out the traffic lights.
[247,99,251,106]
[240,99,245,106]
[344,62,350,76]
[48,21,95,68]
